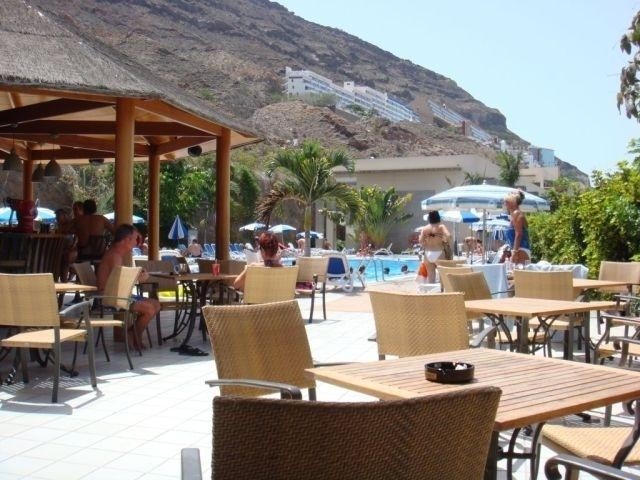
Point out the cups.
[172,263,187,276]
[211,263,221,277]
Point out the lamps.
[2,124,24,172]
[31,134,61,183]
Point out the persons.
[417,209,454,284]
[323,238,332,249]
[231,231,286,291]
[502,192,532,267]
[94,223,162,352]
[348,262,413,277]
[183,238,204,258]
[53,198,149,284]
[297,234,306,253]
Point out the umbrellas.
[420,178,552,265]
[166,213,188,246]
[238,223,270,230]
[295,231,324,238]
[0,206,57,222]
[267,224,298,246]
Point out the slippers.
[171,344,208,355]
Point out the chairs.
[0,235,371,407]
[177,235,640,480]
